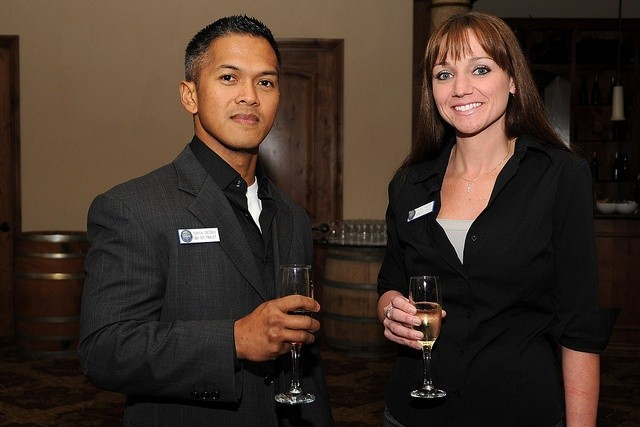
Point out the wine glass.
[274,263,316,406]
[409,276,446,399]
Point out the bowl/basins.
[616,203,638,215]
[598,204,615,213]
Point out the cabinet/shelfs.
[15,232,91,367]
[314,243,391,354]
[592,215,640,358]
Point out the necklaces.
[452,140,511,194]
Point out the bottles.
[590,151,599,183]
[612,152,621,182]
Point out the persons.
[376,11,602,427]
[386,306,393,320]
[77,13,334,427]
